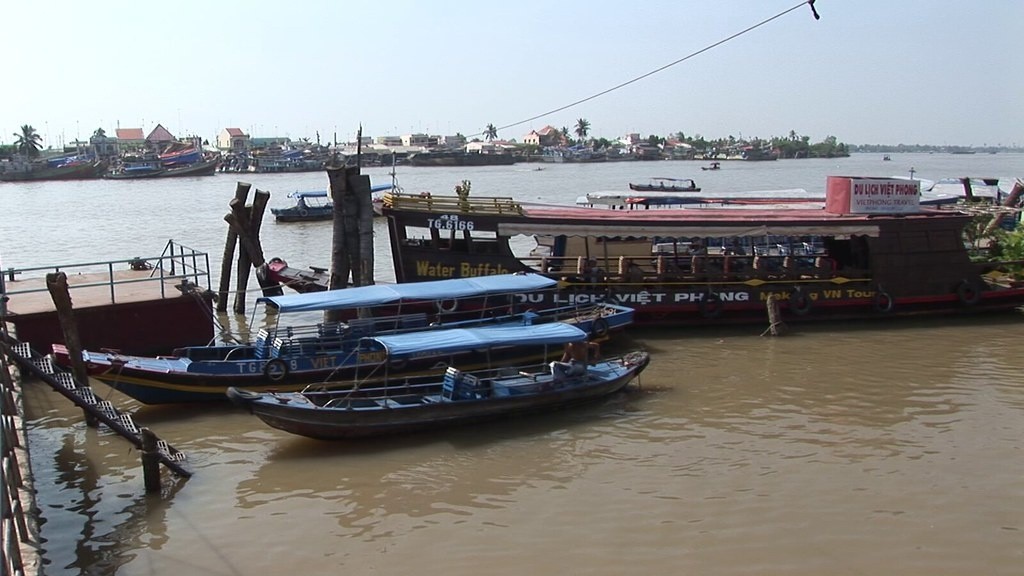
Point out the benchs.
[374,399,402,406]
[539,254,829,279]
[420,367,486,404]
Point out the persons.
[550,338,601,384]
[684,240,707,272]
[660,182,665,190]
[728,240,744,256]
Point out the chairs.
[252,328,284,358]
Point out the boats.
[408,150,517,167]
[884,154,891,161]
[269,184,398,223]
[701,162,720,170]
[1,142,341,181]
[383,173,1024,329]
[541,142,635,163]
[51,271,653,441]
